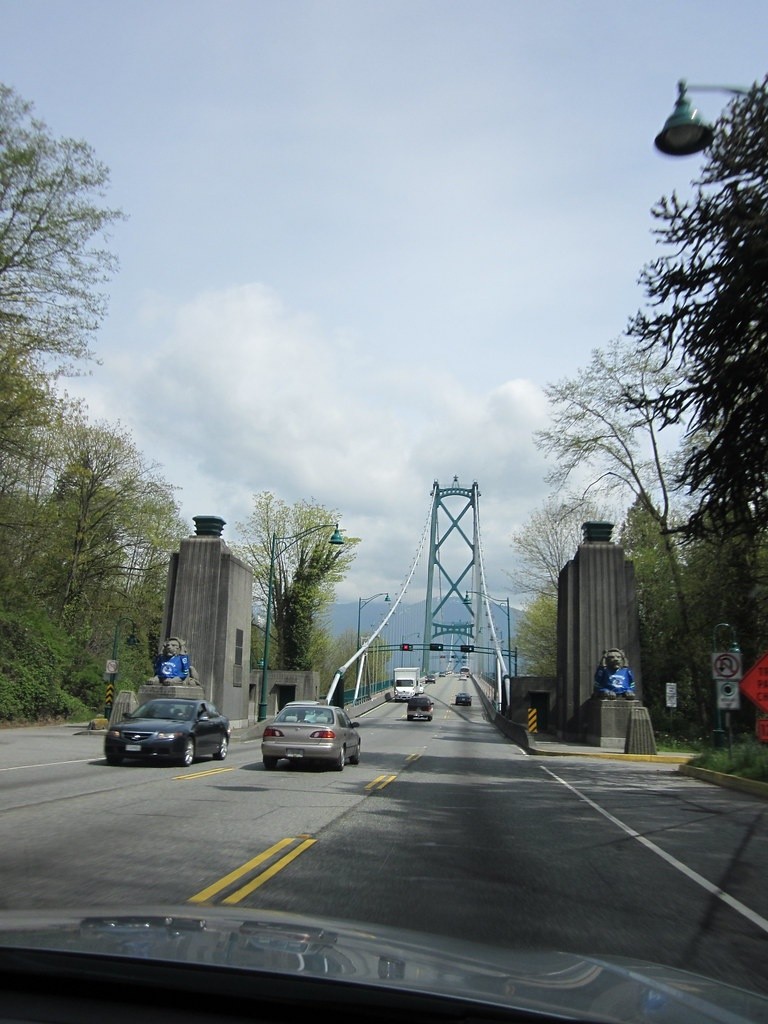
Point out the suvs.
[426,675,435,684]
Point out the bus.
[460,666,470,677]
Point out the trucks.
[394,667,420,702]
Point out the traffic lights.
[403,644,409,650]
[461,645,474,652]
[430,644,443,651]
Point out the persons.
[296,710,311,723]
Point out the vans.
[407,696,434,721]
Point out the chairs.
[315,717,328,724]
[156,705,172,718]
[285,716,297,723]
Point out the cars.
[280,701,323,723]
[262,705,361,770]
[104,696,229,767]
[455,692,472,706]
[460,675,467,681]
[439,671,452,677]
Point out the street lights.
[462,591,511,677]
[258,523,344,721]
[355,593,391,680]
[106,617,141,719]
